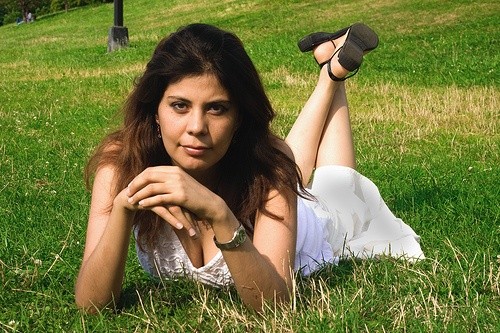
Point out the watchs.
[213,221,247,250]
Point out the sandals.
[297,26,351,70]
[327,22,379,82]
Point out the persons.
[74,23,426,316]
[16,10,32,25]
